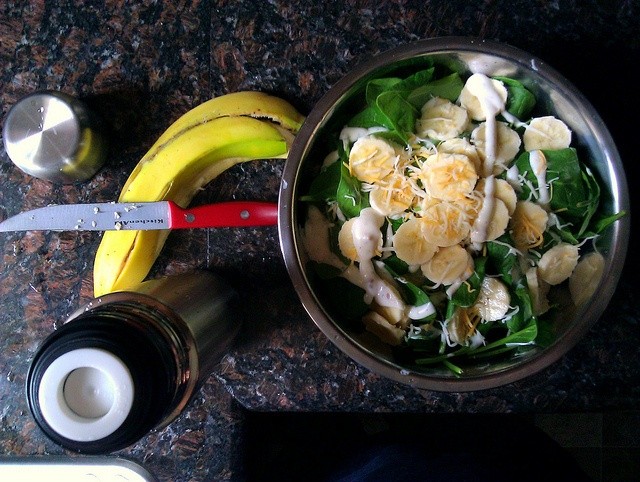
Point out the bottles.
[23,265,243,458]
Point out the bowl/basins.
[276,34,631,393]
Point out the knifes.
[0,199,278,234]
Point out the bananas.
[339,73,603,349]
[90,89,297,300]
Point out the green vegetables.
[301,67,629,374]
